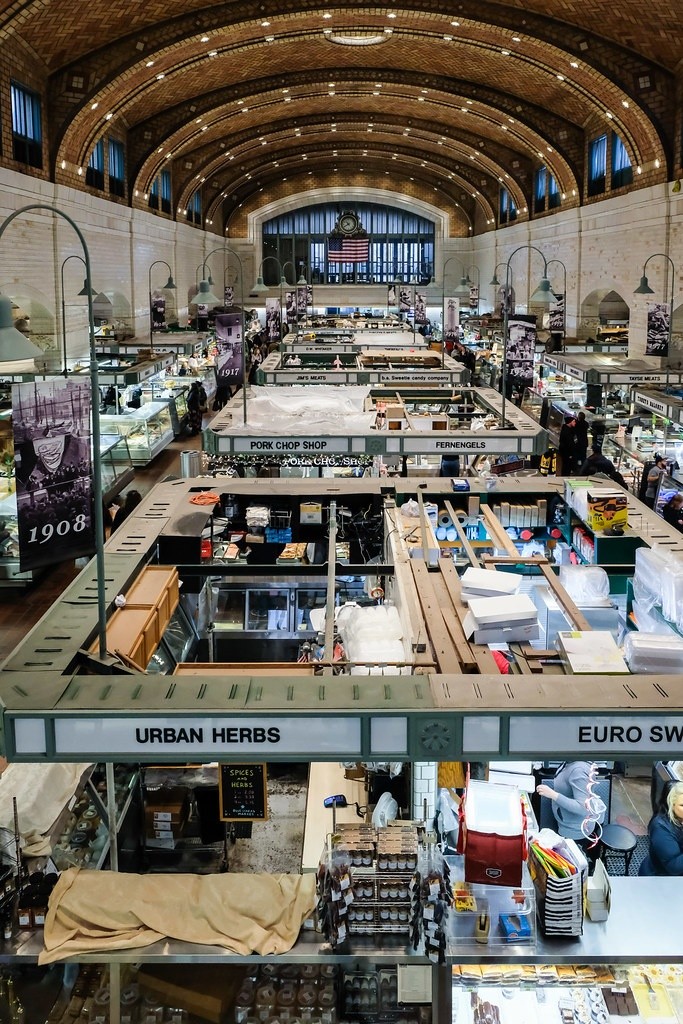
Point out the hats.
[655,455,668,464]
[564,416,575,424]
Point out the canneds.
[58,805,101,862]
[346,850,416,921]
[343,974,398,1008]
[16,872,60,929]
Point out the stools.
[597,825,638,876]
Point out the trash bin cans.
[180,450,201,478]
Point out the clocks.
[339,213,359,233]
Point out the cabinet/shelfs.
[339,870,413,933]
[88,561,181,670]
[552,497,641,566]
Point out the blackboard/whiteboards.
[218,762,268,821]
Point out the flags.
[327,237,369,262]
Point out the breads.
[451,965,683,1024]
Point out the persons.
[246,322,289,382]
[105,387,121,408]
[179,356,197,376]
[536,760,601,873]
[649,780,683,876]
[214,385,233,411]
[449,345,531,405]
[186,381,207,437]
[110,490,142,536]
[559,412,683,532]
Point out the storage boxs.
[138,963,245,1024]
[448,888,538,946]
[459,566,630,675]
[489,501,600,562]
[146,799,183,840]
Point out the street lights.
[501,245,559,427]
[193,262,240,332]
[465,265,480,317]
[190,247,247,425]
[61,256,99,378]
[490,262,513,314]
[392,263,438,345]
[531,258,567,354]
[148,261,178,348]
[441,257,471,387]
[635,253,675,392]
[250,257,309,369]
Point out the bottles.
[53,780,107,867]
[88,964,338,1023]
[4,921,13,941]
[345,850,416,936]
[343,974,415,1023]
[18,870,58,928]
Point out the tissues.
[498,913,530,944]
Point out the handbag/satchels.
[212,401,219,411]
[528,833,588,935]
